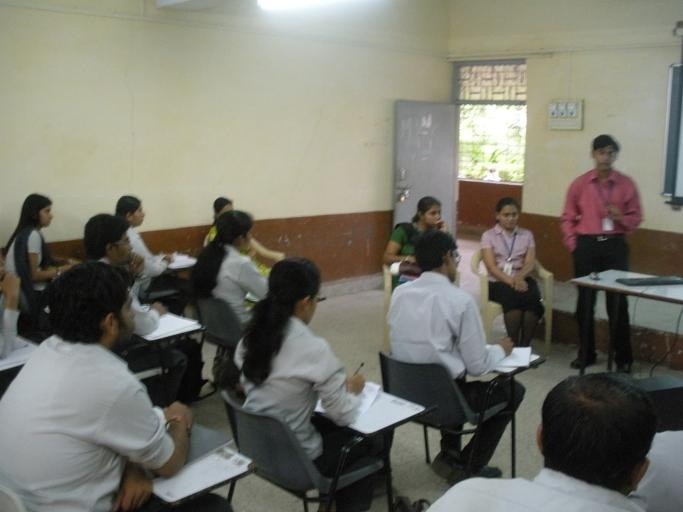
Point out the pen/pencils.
[509,329,514,337]
[352,362,365,377]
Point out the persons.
[631,430,683,512]
[425,372,658,512]
[381,196,449,294]
[1,193,395,511]
[560,134,642,373]
[385,229,526,487]
[480,197,545,348]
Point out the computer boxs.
[638,375,683,432]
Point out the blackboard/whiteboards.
[660,63,683,205]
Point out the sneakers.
[430,450,462,477]
[446,461,502,484]
[569,351,599,370]
[616,361,632,375]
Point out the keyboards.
[615,274,683,286]
